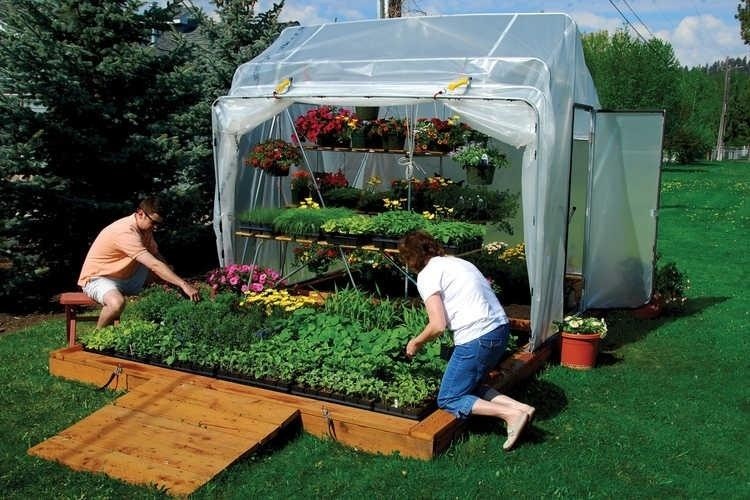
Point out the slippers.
[499,403,537,452]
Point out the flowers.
[552,312,608,339]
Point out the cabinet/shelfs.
[236,145,482,300]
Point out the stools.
[60,292,119,348]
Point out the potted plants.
[633,254,687,317]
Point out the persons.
[77,195,201,330]
[398,230,536,451]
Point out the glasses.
[141,208,164,228]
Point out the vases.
[560,332,600,369]
